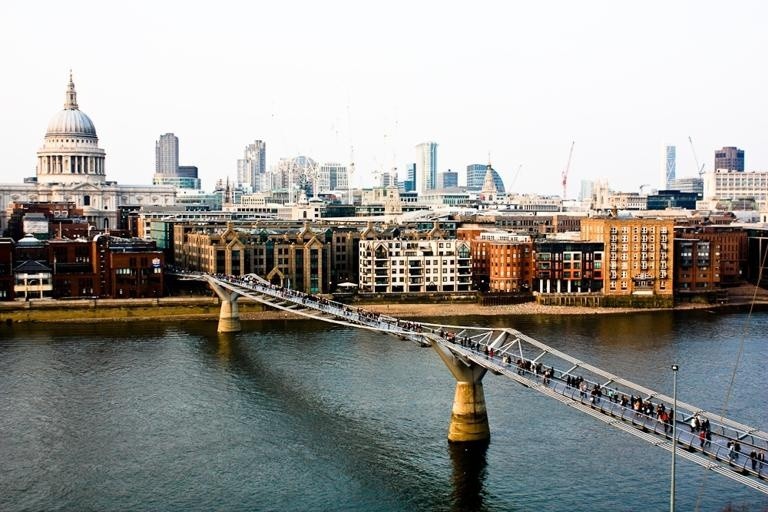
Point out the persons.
[165,264,245,285]
[566,375,674,433]
[516,359,555,386]
[343,306,424,338]
[245,275,329,309]
[690,414,764,470]
[431,328,512,367]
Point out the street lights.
[153,266,162,305]
[670,364,678,512]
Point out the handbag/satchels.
[590,397,593,401]
